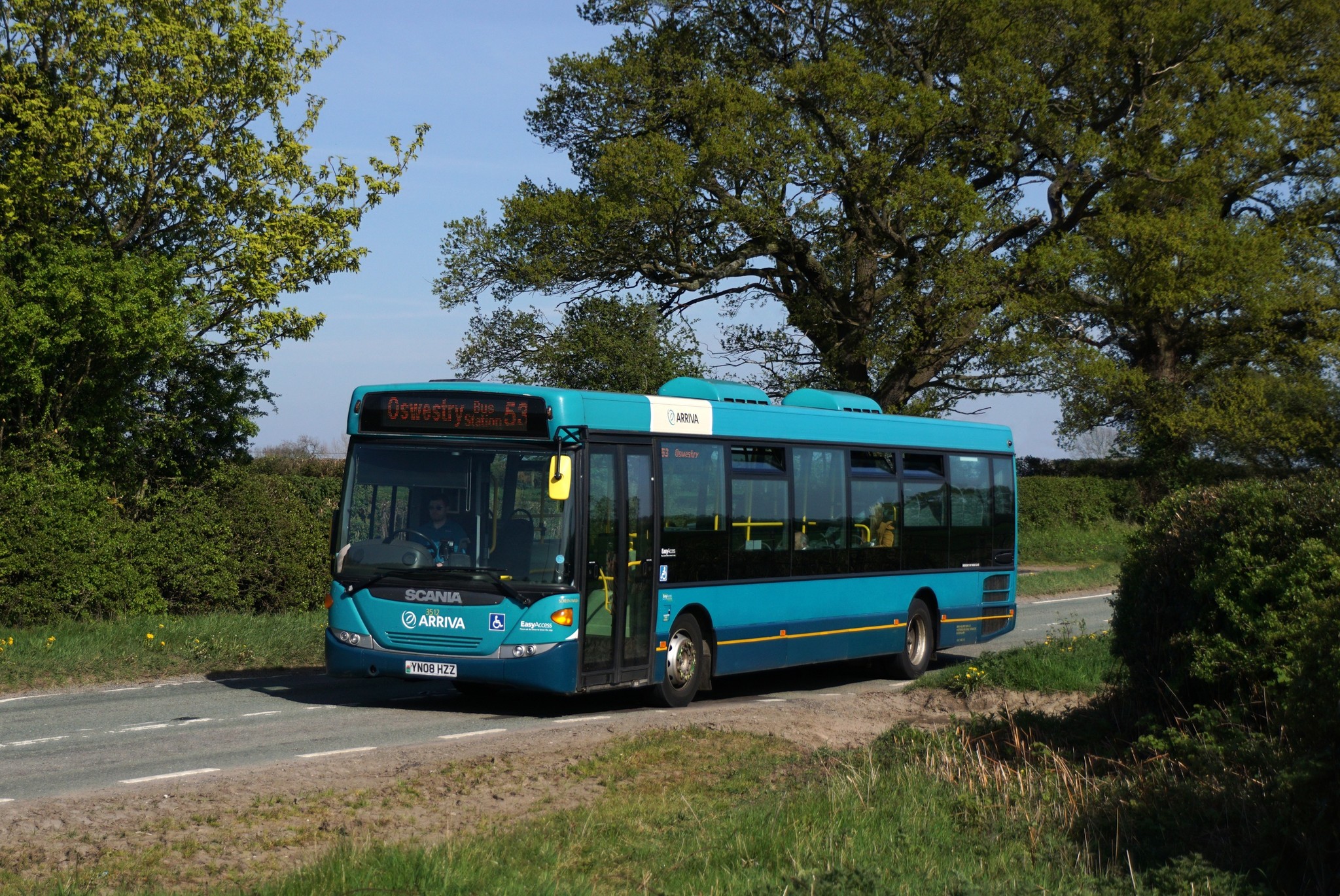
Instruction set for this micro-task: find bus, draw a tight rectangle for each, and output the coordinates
[322,376,1018,708]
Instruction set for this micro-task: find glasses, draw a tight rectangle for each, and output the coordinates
[428,504,445,511]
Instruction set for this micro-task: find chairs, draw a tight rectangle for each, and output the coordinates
[738,486,1004,550]
[447,506,533,578]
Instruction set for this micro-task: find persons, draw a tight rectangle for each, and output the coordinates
[410,493,468,556]
[793,532,813,550]
[858,500,895,547]
